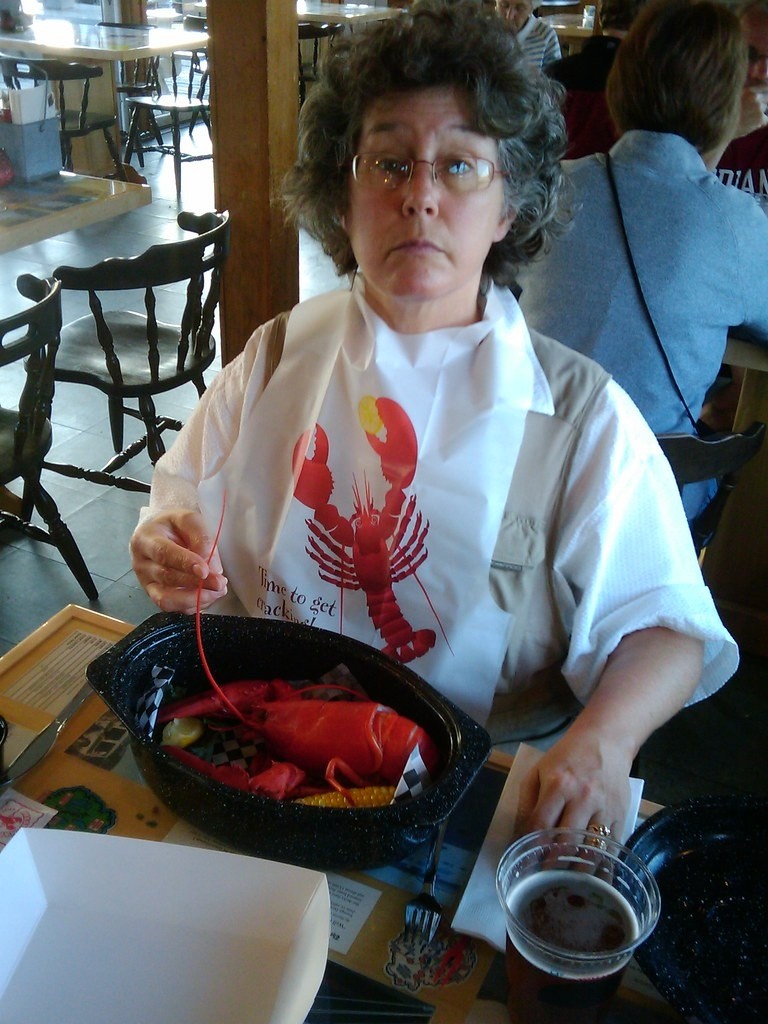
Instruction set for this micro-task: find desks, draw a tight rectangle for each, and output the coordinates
[297,0,404,70]
[0,19,210,188]
[0,170,153,518]
[536,13,628,55]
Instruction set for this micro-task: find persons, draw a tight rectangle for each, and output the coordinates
[512,0,768,547]
[128,0,739,884]
[494,0,562,76]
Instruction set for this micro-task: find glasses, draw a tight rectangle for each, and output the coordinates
[348,152,513,192]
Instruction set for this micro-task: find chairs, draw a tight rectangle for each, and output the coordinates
[24,210,232,493]
[0,52,129,185]
[0,274,99,601]
[97,23,163,169]
[124,13,213,192]
[656,420,768,564]
[298,21,347,108]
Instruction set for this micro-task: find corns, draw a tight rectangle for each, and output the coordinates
[289,785,398,809]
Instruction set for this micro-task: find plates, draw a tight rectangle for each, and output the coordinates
[612,791,768,1023]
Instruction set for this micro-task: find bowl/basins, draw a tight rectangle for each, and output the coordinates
[85,612,491,872]
[495,828,661,980]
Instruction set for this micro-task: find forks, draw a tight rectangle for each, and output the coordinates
[401,817,449,948]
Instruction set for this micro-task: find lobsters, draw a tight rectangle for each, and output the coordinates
[156,676,442,804]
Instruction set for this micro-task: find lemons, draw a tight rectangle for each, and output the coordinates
[161,717,204,749]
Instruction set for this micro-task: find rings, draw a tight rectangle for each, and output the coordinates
[585,825,610,857]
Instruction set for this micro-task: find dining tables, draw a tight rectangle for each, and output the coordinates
[1,605,673,1023]
